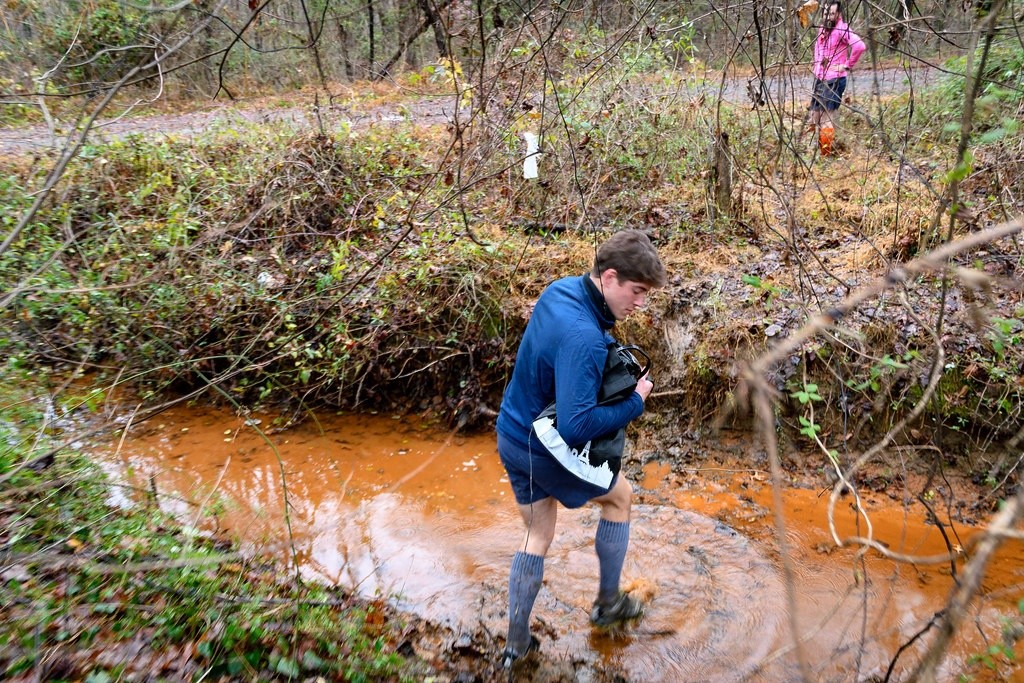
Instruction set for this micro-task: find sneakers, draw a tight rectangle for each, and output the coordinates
[590,591,645,626]
[502,636,540,667]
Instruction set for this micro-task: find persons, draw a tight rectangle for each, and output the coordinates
[811,0,866,156]
[495,229,665,668]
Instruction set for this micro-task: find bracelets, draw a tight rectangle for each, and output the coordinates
[844,66,849,71]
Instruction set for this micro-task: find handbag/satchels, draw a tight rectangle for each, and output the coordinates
[530,343,651,490]
